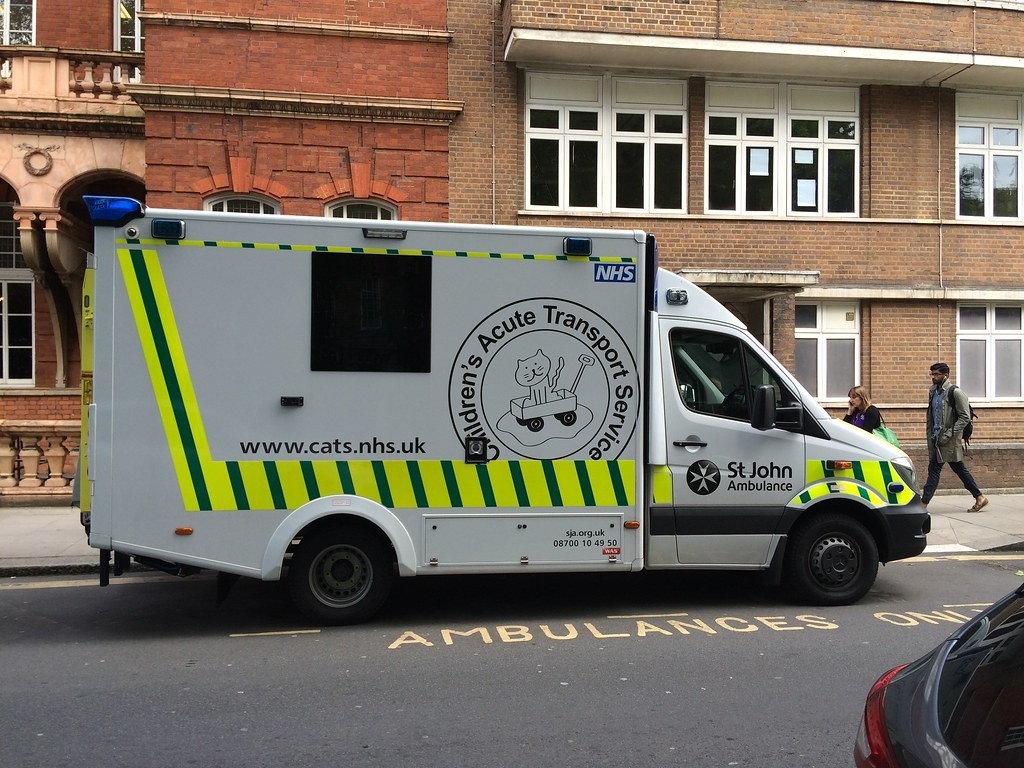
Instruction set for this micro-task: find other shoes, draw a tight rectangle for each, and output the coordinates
[967,498,989,513]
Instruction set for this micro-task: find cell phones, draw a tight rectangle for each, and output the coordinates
[849,401,853,406]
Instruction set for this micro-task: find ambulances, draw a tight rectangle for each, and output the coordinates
[73,194,932,629]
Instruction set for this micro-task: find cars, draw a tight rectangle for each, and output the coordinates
[851,583,1024,768]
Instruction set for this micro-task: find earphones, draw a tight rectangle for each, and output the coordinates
[943,376,947,380]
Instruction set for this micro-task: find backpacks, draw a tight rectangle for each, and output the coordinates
[948,385,978,452]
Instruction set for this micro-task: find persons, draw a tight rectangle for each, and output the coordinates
[842,386,881,434]
[920,363,989,512]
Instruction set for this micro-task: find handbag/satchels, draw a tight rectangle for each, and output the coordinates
[866,406,901,449]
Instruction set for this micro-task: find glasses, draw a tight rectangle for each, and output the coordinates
[929,372,945,376]
